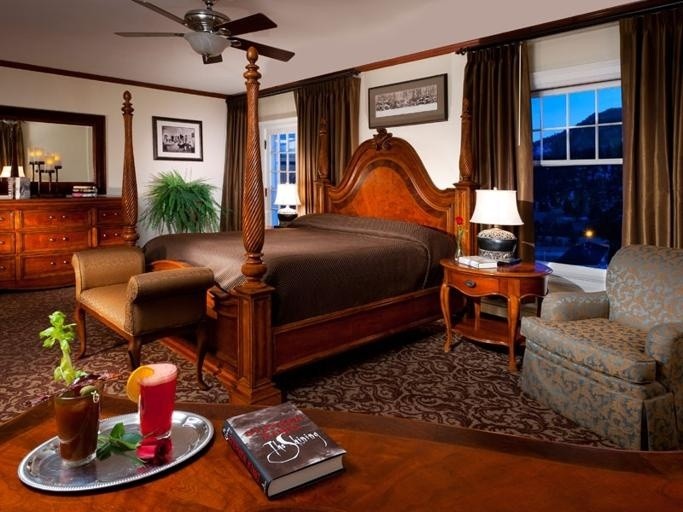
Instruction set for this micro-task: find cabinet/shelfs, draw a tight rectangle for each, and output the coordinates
[0,196,121,289]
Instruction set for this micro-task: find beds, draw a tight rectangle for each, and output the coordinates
[121,44,478,408]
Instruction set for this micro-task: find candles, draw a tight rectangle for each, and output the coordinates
[44,156,54,171]
[34,148,44,161]
[52,153,62,167]
[27,147,35,163]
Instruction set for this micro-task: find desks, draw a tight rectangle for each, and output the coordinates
[438,257,553,371]
[0,394,683,512]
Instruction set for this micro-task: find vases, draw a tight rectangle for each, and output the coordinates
[455,230,464,263]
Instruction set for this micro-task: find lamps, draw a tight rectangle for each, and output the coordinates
[183,33,231,57]
[0,165,26,177]
[274,184,303,222]
[469,186,524,264]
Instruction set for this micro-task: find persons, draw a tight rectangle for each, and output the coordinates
[162,134,194,152]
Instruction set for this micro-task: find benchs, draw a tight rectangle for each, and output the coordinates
[70,245,219,391]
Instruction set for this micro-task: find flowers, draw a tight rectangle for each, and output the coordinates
[455,216,466,255]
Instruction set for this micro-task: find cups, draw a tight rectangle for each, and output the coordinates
[53,382,106,468]
[138,363,179,441]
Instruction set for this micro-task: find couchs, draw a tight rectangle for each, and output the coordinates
[518,245,683,451]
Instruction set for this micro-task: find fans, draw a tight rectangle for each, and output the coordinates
[114,0,294,64]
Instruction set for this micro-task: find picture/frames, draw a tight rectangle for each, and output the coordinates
[152,116,203,162]
[368,73,448,129]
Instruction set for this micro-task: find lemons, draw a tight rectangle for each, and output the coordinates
[126,366,154,404]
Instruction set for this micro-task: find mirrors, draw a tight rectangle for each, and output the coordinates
[0,105,106,197]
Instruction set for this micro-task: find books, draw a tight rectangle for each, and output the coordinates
[456,255,497,268]
[8,177,30,200]
[72,185,98,198]
[221,400,347,498]
